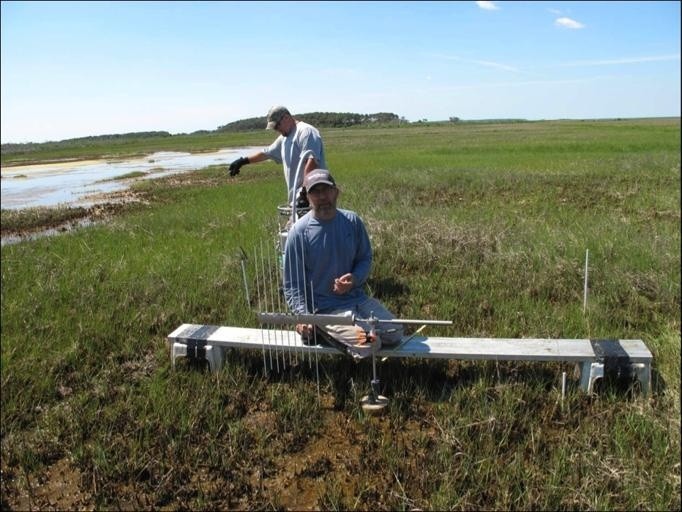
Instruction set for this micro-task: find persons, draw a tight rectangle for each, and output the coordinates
[228,106,327,219]
[280,166,403,396]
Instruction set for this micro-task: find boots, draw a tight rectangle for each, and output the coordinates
[300,322,349,358]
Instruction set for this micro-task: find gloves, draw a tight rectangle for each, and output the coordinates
[294,186,311,209]
[228,156,251,178]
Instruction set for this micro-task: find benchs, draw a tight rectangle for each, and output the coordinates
[167,323,655,399]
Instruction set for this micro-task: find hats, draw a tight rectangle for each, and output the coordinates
[301,168,336,194]
[264,106,290,130]
